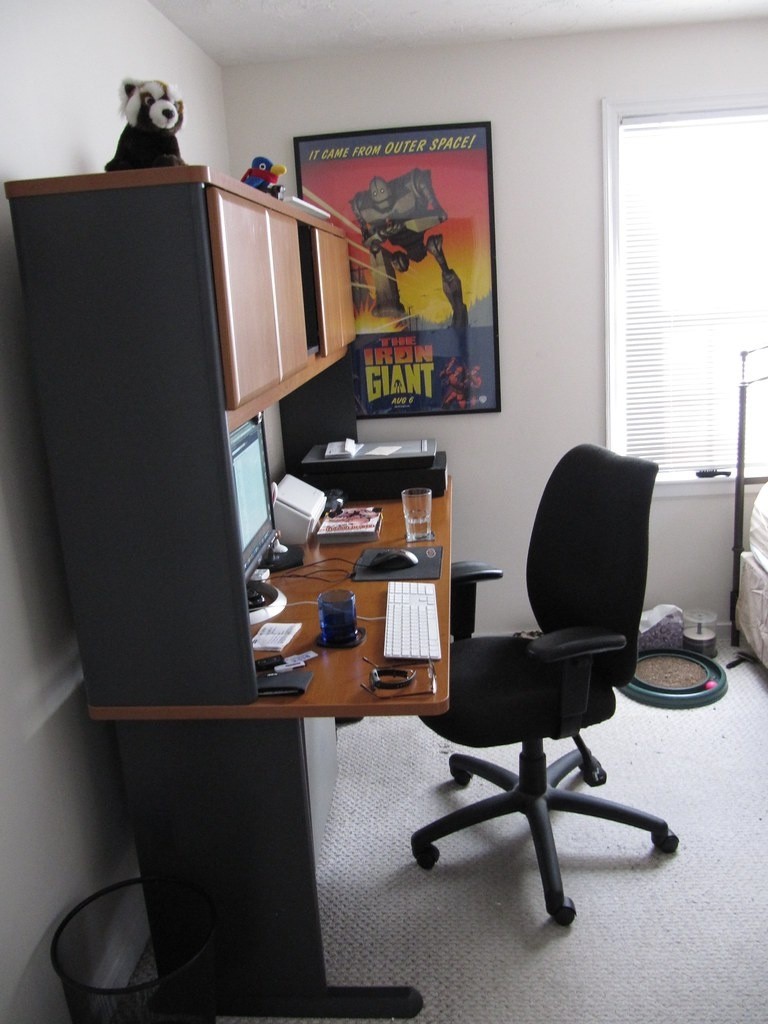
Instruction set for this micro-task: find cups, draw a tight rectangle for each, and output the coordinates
[318,589,359,643]
[401,488,432,540]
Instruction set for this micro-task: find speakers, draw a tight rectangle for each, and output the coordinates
[272,474,327,549]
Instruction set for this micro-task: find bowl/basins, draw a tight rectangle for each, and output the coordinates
[634,654,710,693]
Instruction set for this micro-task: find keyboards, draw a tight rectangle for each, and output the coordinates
[383,581,441,660]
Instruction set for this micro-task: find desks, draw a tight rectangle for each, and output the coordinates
[89,473,453,1020]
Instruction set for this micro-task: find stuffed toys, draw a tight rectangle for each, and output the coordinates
[241,157,287,201]
[105,80,183,172]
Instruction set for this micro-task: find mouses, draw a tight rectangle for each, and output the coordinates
[370,549,418,571]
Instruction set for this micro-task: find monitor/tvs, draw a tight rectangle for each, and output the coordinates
[228,411,279,613]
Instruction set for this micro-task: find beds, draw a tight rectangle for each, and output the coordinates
[734,482,768,669]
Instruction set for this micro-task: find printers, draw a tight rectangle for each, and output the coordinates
[301,439,448,499]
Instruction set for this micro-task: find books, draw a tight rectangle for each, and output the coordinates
[317,504,384,544]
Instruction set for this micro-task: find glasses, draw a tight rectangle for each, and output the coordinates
[360,656,437,699]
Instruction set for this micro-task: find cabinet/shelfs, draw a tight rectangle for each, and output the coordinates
[6,167,357,706]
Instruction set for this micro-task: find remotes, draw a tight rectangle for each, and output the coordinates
[696,470,731,477]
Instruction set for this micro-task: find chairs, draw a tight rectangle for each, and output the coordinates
[410,444,680,924]
